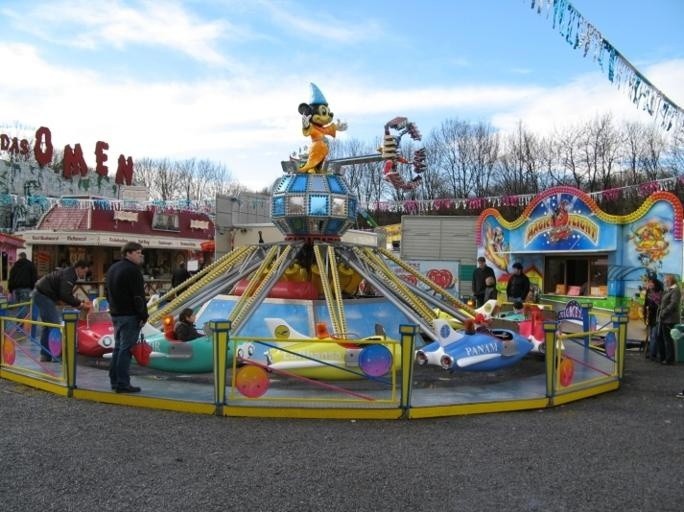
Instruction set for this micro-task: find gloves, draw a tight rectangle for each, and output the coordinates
[77,300,93,311]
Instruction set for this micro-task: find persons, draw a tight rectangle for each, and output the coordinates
[642,275,681,364]
[32,259,92,362]
[504,263,531,302]
[191,260,208,276]
[7,251,37,331]
[174,307,198,343]
[104,242,150,393]
[511,301,525,316]
[471,256,496,306]
[474,276,498,305]
[171,260,193,296]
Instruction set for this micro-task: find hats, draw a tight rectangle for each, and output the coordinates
[648,272,658,279]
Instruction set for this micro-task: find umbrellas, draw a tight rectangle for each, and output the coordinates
[128,333,154,368]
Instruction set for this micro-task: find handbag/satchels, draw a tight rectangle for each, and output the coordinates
[131,340,152,365]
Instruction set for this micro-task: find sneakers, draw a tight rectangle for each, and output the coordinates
[39,353,54,361]
[112,380,142,393]
[658,359,674,364]
